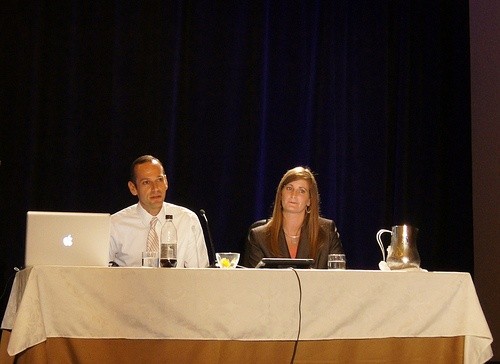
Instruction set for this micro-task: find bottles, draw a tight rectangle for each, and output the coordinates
[160,215,178,268]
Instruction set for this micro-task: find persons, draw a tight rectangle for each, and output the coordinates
[246,167,345,269]
[108,155,211,269]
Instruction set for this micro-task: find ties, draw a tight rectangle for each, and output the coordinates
[146,217,159,268]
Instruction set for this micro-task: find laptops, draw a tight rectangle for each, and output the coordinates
[25,212,112,266]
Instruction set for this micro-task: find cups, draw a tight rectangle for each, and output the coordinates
[142,251,159,267]
[327,254,346,269]
[215,253,240,269]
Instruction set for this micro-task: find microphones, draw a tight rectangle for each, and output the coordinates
[199,210,218,269]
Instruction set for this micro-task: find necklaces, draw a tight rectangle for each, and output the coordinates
[284,229,300,238]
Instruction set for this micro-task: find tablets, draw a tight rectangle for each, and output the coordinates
[260,257,315,268]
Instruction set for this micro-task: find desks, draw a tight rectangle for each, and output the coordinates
[0,264,493,364]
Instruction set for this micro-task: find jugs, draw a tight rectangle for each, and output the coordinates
[376,225,421,270]
